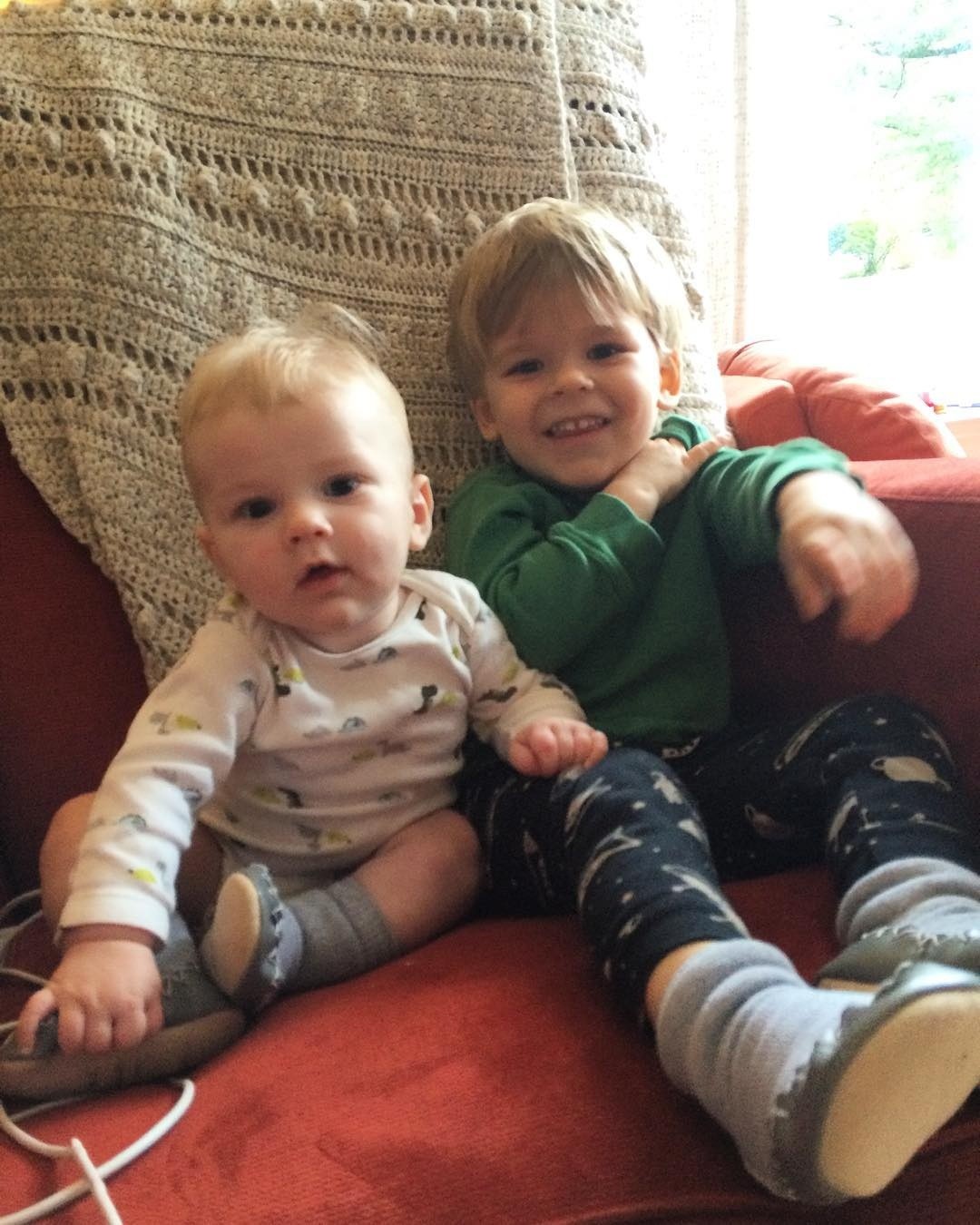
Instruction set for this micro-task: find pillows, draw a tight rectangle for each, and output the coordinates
[716,338,965,462]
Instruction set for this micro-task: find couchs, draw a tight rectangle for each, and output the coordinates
[0,0,980,1225]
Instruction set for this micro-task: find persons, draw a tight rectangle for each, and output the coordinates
[441,194,979,1208]
[0,301,609,1102]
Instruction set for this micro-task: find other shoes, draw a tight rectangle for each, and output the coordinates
[0,942,243,1101]
[818,926,980,996]
[778,964,979,1210]
[200,861,280,1011]
